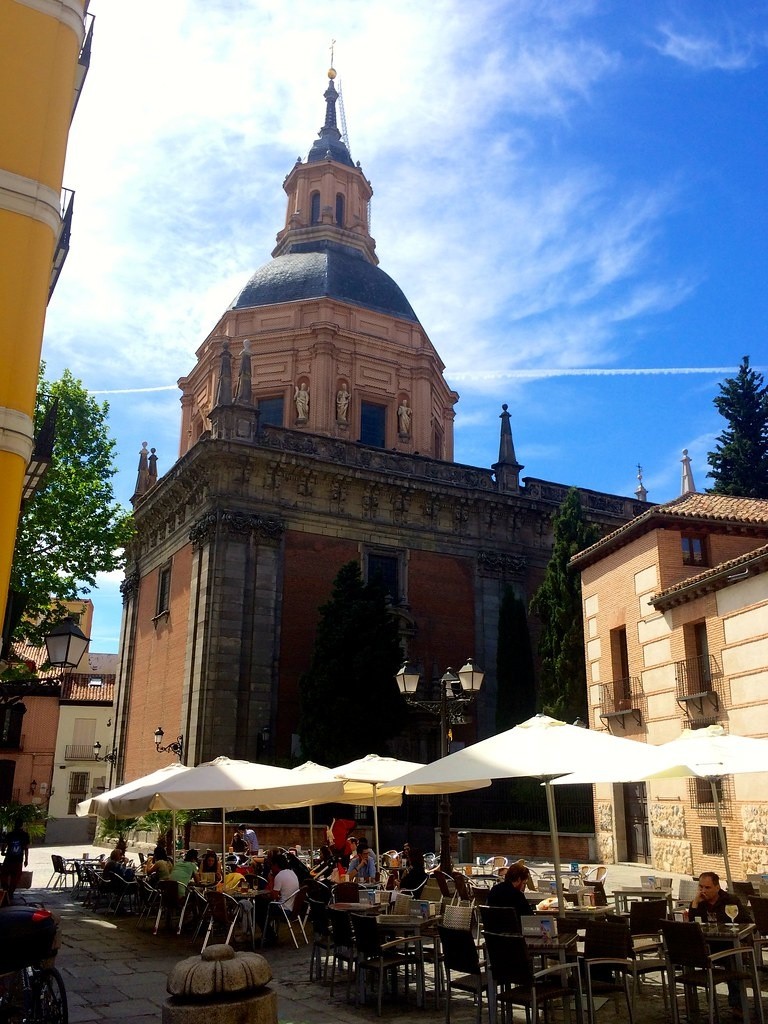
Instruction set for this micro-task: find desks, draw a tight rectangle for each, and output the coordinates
[658,923,759,1024]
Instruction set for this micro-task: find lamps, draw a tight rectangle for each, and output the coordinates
[30,779,37,795]
[0,616,89,715]
[256,726,271,746]
[573,717,587,729]
[154,727,183,761]
[93,741,117,768]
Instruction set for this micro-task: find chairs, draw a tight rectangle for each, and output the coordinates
[45,850,767,1024]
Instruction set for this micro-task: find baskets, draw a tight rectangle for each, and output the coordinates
[394,893,413,915]
[443,894,475,931]
[376,891,391,904]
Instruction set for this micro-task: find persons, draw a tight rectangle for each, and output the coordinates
[101,822,427,948]
[0,817,29,904]
[397,398,413,435]
[485,863,534,927]
[685,872,759,1021]
[293,382,310,419]
[337,382,351,421]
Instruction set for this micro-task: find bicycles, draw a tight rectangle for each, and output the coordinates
[0,895,68,1024]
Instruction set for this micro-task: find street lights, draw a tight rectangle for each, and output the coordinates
[392,657,486,872]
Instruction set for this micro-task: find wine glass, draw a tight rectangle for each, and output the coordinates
[253,879,259,893]
[725,905,740,932]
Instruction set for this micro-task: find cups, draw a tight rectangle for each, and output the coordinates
[83,853,89,859]
[694,917,702,924]
[566,902,574,908]
[258,849,263,857]
[241,881,250,894]
[583,896,591,906]
[295,844,301,854]
[707,912,717,927]
[200,872,216,884]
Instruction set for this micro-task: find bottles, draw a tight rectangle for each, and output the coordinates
[143,864,147,874]
[307,848,312,858]
[226,846,237,873]
[132,863,136,873]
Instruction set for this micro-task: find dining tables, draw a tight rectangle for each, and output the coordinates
[541,871,581,884]
[612,888,673,921]
[382,866,406,891]
[235,890,272,934]
[461,875,500,889]
[329,903,390,933]
[452,863,485,887]
[379,914,443,1007]
[330,883,381,905]
[485,933,578,1024]
[532,906,616,956]
[65,859,101,889]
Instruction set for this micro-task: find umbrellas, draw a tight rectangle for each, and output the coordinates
[72,712,494,870]
[377,712,661,920]
[541,725,768,894]
